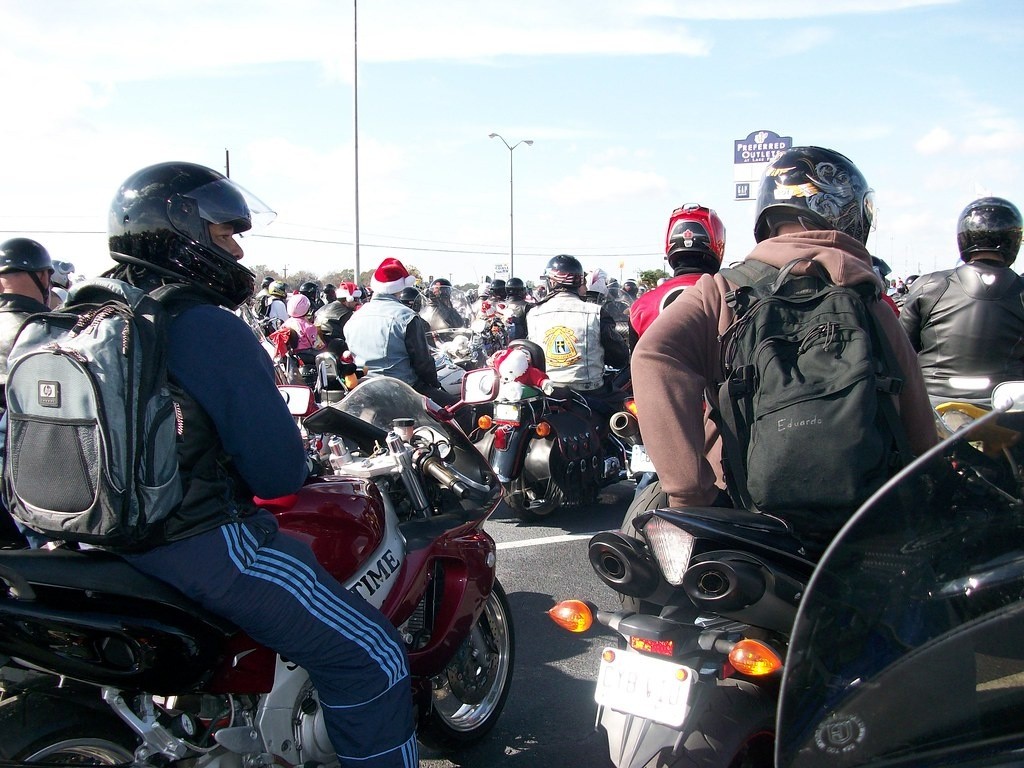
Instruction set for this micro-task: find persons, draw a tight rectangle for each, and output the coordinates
[628,204,727,356]
[0,237,76,408]
[400,267,671,361]
[630,146,940,508]
[870,255,921,316]
[899,197,1024,408]
[342,258,473,437]
[52,162,419,768]
[528,256,629,416]
[252,276,374,352]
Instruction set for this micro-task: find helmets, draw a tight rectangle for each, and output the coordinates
[48,259,76,289]
[299,281,318,296]
[665,201,727,273]
[269,279,288,297]
[620,282,639,295]
[752,145,878,249]
[505,278,524,296]
[488,279,506,290]
[539,254,585,289]
[108,161,280,312]
[0,238,56,275]
[956,197,1023,266]
[400,287,422,313]
[429,278,452,289]
[323,283,337,296]
[261,276,274,288]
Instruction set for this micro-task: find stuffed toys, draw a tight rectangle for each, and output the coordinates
[486,346,554,402]
[436,335,468,358]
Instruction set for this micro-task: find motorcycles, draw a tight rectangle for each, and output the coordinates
[0,367,516,768]
[542,404,1024,768]
[255,313,375,468]
[481,339,647,517]
[605,396,733,625]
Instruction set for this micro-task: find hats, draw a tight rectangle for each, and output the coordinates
[607,278,622,289]
[370,256,415,295]
[287,294,311,317]
[335,282,362,303]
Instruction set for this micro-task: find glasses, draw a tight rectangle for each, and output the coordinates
[354,297,360,302]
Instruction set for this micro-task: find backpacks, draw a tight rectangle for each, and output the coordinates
[5,277,205,553]
[252,295,272,320]
[716,261,938,550]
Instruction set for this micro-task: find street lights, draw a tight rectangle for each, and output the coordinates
[489,132,533,280]
